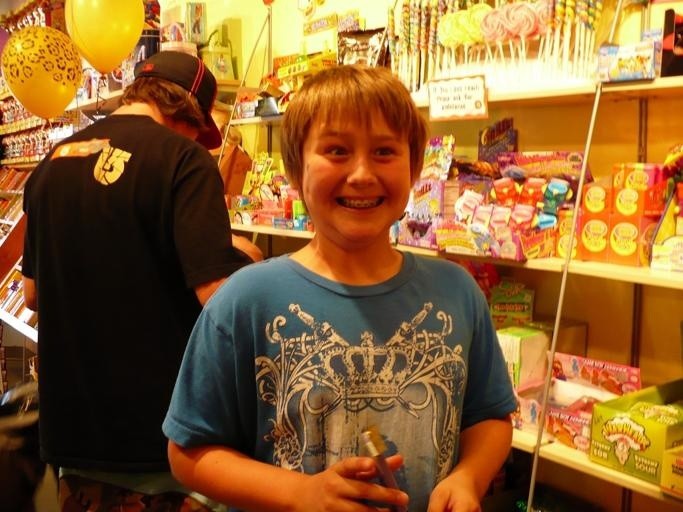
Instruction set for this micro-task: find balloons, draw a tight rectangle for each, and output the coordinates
[64,0,145,74]
[0,26,82,119]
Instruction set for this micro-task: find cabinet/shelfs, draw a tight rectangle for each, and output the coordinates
[0,89,126,169]
[230,76,683,512]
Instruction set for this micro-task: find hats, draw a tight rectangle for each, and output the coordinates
[135,52,222,150]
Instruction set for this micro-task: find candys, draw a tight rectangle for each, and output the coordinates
[384,1,602,94]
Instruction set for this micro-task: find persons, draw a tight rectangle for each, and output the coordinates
[21,51,264,512]
[160,67,519,512]
